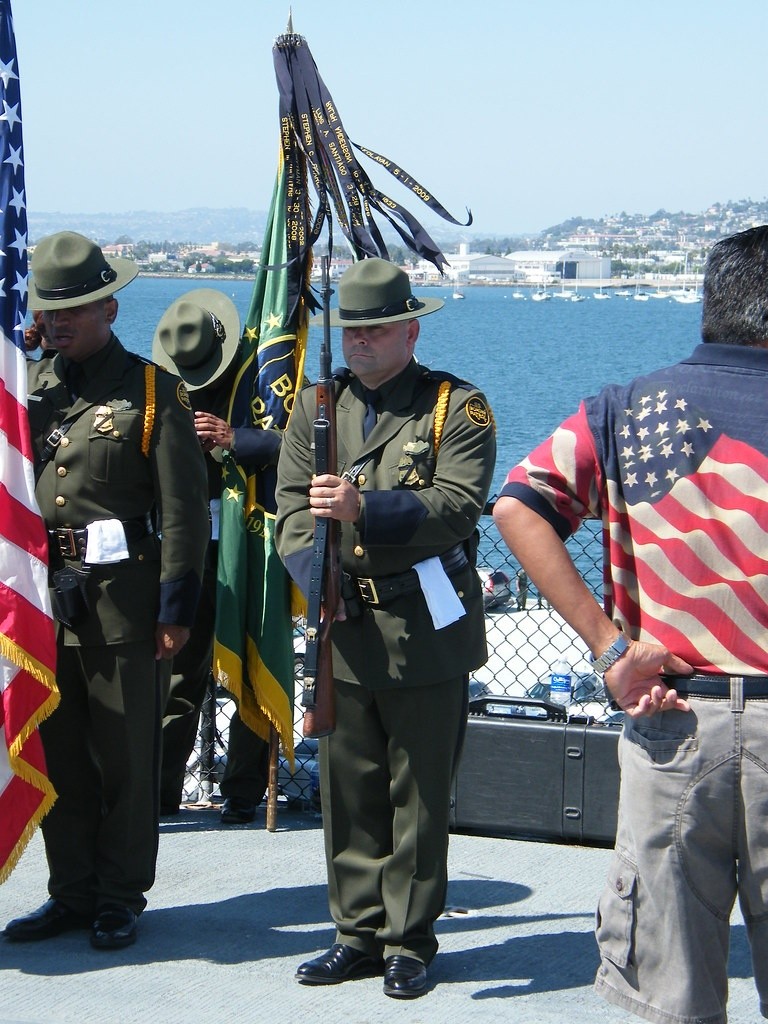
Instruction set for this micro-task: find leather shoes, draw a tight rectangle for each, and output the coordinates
[4,898,92,940]
[294,944,384,983]
[382,955,427,995]
[218,797,257,823]
[90,904,137,948]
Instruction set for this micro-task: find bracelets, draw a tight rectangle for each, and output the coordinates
[588,631,632,674]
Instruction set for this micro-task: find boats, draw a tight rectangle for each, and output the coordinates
[615,291,632,296]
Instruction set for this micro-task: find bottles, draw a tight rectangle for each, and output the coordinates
[550,655,572,724]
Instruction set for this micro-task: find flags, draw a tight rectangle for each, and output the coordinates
[210,161,303,774]
[0,0,62,888]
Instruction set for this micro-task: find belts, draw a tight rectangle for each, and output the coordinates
[658,670,767,700]
[343,541,471,606]
[47,512,154,560]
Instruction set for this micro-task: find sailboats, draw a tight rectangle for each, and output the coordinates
[571,262,586,303]
[634,254,649,301]
[452,276,465,299]
[592,260,611,299]
[512,249,551,301]
[646,252,704,303]
[553,261,575,298]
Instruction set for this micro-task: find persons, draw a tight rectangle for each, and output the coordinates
[493,222,768,1023]
[515,565,530,612]
[536,591,551,609]
[273,257,498,1000]
[154,286,312,828]
[5,230,212,952]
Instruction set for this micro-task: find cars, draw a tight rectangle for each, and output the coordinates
[478,568,510,612]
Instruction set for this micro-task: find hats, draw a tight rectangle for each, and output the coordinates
[151,287,241,391]
[26,230,139,310]
[307,257,445,327]
